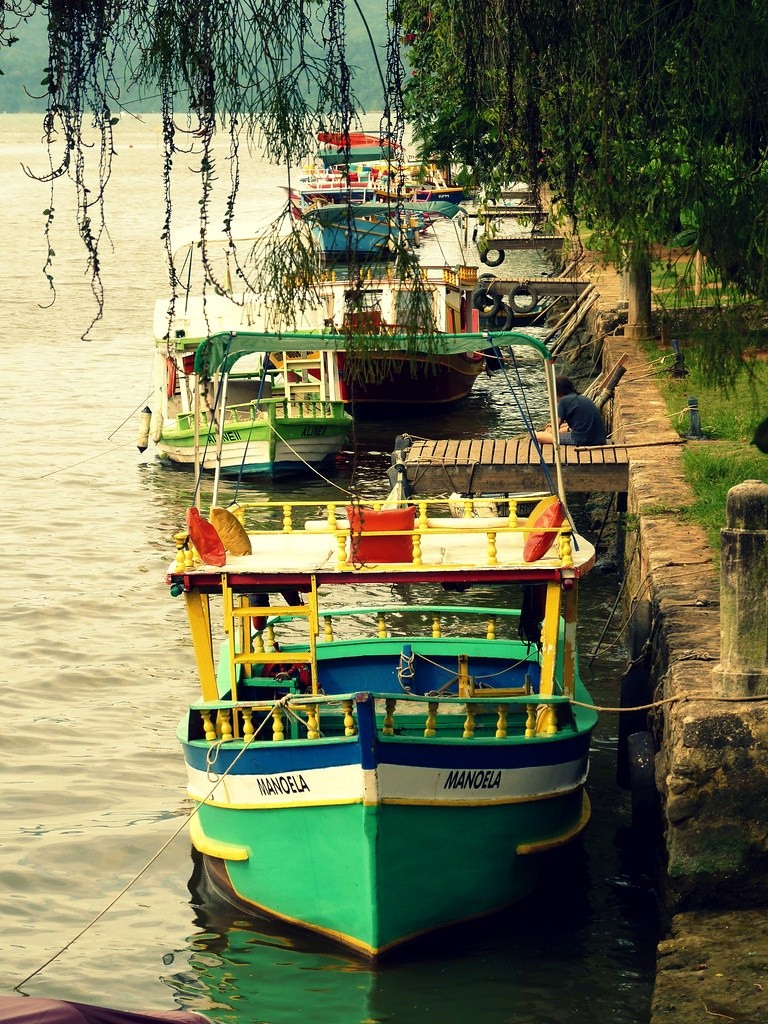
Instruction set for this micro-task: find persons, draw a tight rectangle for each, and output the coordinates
[536,375,606,445]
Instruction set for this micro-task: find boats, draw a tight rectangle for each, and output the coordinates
[150,300,352,482]
[165,330,599,964]
[279,130,477,269]
[270,266,486,406]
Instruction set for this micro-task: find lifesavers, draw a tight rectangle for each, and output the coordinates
[482,246,506,267]
[473,283,538,333]
